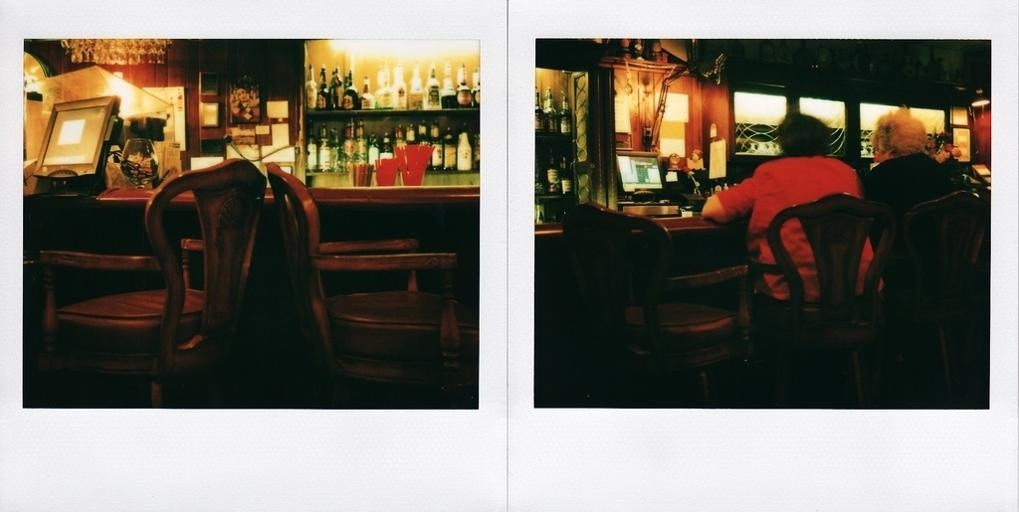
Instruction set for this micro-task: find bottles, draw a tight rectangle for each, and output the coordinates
[688,170,706,197]
[306,119,479,171]
[593,38,663,62]
[535,88,570,135]
[535,199,545,224]
[535,146,572,197]
[304,59,481,109]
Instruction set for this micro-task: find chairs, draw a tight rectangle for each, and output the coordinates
[267,164,478,409]
[564,202,748,407]
[897,190,989,409]
[737,193,897,409]
[36,162,266,408]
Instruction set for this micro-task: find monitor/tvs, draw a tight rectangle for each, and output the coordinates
[615,150,664,203]
[30,94,123,195]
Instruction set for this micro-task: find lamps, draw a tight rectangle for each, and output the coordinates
[971,89,990,108]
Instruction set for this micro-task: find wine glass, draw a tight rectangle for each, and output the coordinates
[66,40,169,65]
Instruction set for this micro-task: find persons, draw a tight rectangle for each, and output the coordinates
[865,107,979,315]
[701,112,885,322]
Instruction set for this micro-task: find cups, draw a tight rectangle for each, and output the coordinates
[118,137,160,190]
[393,143,435,185]
[374,158,397,186]
[352,164,372,187]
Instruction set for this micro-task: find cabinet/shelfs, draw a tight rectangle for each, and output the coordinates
[534,129,574,237]
[305,108,480,177]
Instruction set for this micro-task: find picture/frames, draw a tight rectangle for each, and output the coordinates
[226,70,262,127]
